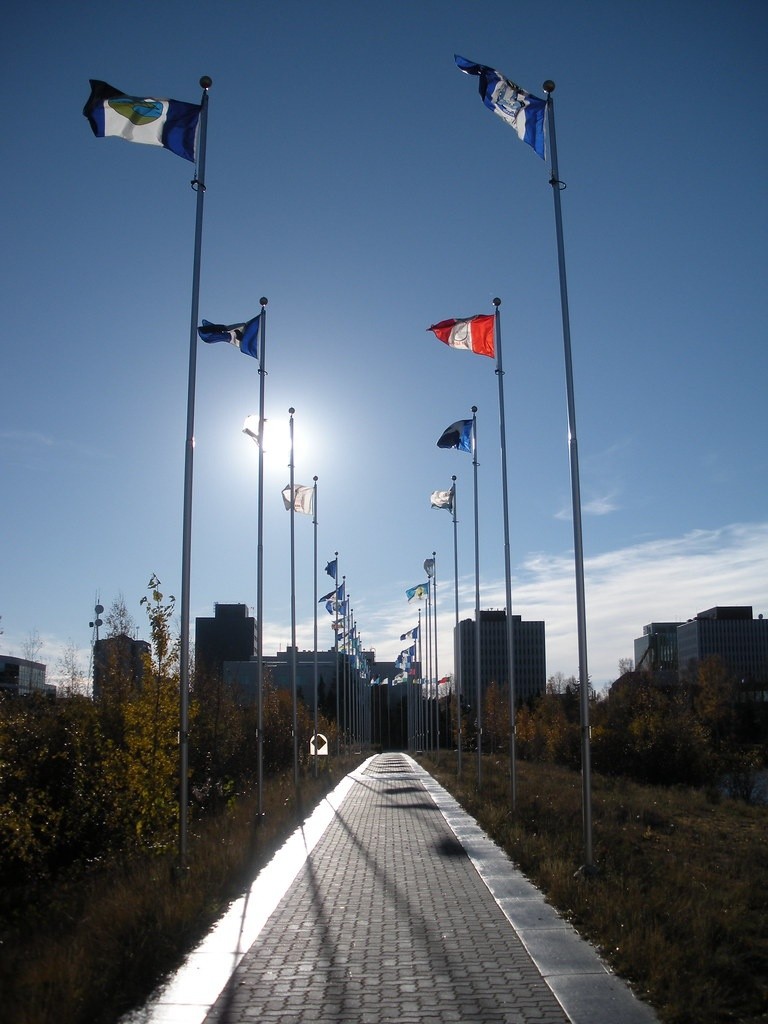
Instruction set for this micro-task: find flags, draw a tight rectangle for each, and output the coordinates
[452,54,546,160]
[427,314,495,359]
[83,79,202,161]
[430,487,454,514]
[199,314,261,359]
[283,483,314,514]
[319,559,451,688]
[437,419,474,454]
